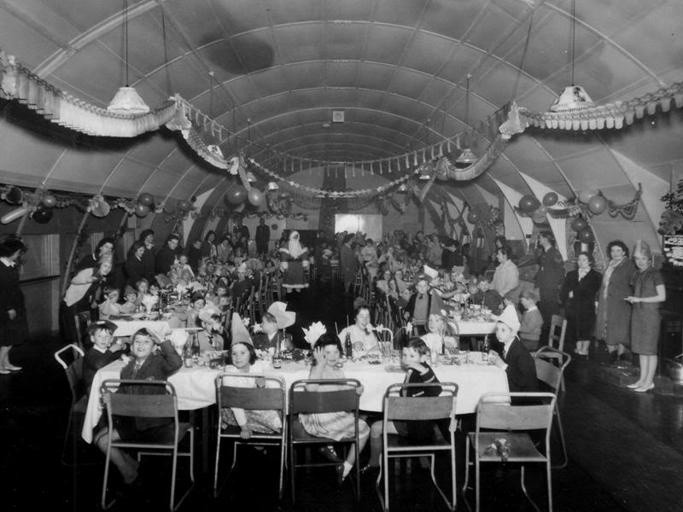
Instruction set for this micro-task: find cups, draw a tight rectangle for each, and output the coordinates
[341,341,401,365]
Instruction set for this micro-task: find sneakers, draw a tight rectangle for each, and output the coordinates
[319,446,339,461]
[336,464,344,488]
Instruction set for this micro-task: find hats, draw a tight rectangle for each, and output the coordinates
[413,273,432,284]
[430,290,441,314]
[231,312,253,347]
[87,320,117,330]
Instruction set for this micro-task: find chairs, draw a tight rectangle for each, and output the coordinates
[100,378,196,509]
[289,378,365,505]
[462,391,558,510]
[374,382,459,511]
[213,372,287,500]
[54,344,90,443]
[534,344,572,469]
[56,255,572,359]
[529,315,568,392]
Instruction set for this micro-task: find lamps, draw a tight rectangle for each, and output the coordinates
[550,0,596,112]
[208,71,292,199]
[397,119,431,192]
[107,0,150,115]
[457,73,477,164]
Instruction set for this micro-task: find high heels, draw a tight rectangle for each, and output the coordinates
[626,382,655,392]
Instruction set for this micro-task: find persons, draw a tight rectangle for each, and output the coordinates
[1,189,665,486]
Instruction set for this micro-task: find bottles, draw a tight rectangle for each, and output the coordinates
[434,333,492,368]
[135,289,184,320]
[459,294,492,322]
[271,331,284,370]
[184,328,201,370]
[344,334,351,357]
